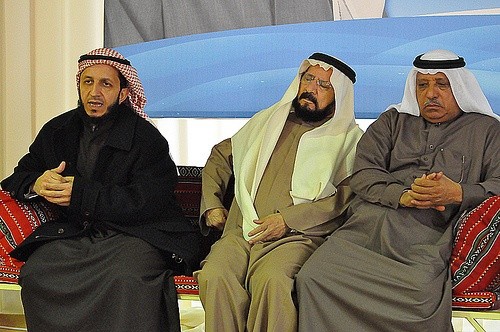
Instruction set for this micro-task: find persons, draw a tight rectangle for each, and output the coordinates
[193,52,366,332]
[297,50,500,332]
[0,49,218,332]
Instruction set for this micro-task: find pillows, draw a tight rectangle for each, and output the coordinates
[1,167,499,313]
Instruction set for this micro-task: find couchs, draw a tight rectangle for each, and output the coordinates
[0,114,499,331]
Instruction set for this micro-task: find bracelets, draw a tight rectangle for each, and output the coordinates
[400,190,408,208]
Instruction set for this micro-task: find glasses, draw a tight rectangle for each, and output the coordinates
[299,73,333,91]
